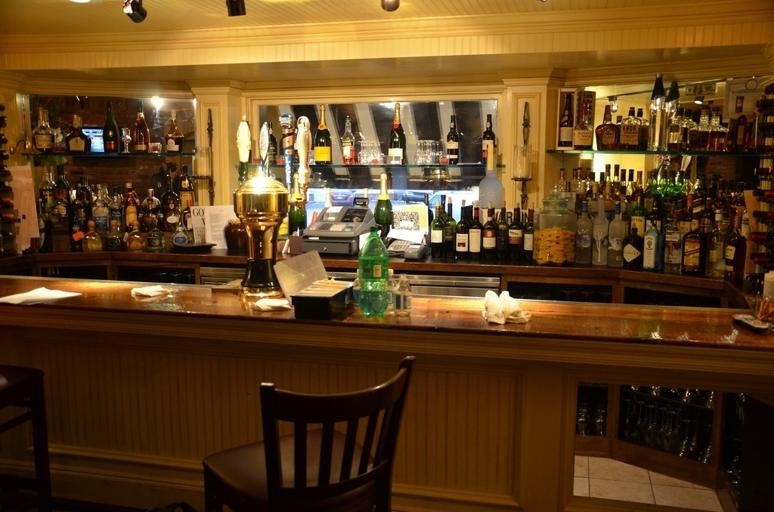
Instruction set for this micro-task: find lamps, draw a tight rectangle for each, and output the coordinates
[121,0,147,23]
[694,96,704,104]
[381,0,400,11]
[226,0,246,16]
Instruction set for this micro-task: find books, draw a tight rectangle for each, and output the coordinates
[191,206,205,244]
[203,206,238,249]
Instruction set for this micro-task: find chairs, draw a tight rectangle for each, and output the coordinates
[201,354,415,511]
[1,364,53,511]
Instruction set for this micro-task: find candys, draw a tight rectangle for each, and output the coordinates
[536,227,574,266]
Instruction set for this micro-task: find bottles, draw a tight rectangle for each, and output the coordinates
[617,169,629,198]
[667,108,688,152]
[582,167,591,187]
[144,190,160,208]
[481,114,496,164]
[342,116,355,164]
[64,115,87,153]
[508,204,524,264]
[599,164,614,203]
[662,172,678,217]
[124,191,139,232]
[423,195,433,226]
[177,166,195,208]
[705,176,753,209]
[614,115,622,129]
[608,202,629,268]
[265,123,275,163]
[289,176,305,238]
[70,188,89,233]
[678,172,688,193]
[160,178,180,231]
[568,168,584,194]
[705,209,726,279]
[496,201,508,264]
[43,109,57,151]
[114,185,124,205]
[37,166,52,210]
[680,109,697,151]
[395,274,410,315]
[586,173,598,212]
[387,103,406,165]
[55,166,68,200]
[533,189,576,266]
[386,269,397,315]
[166,119,182,153]
[374,174,394,239]
[725,207,747,282]
[619,107,641,149]
[356,228,388,317]
[107,188,122,231]
[612,164,620,197]
[430,203,443,258]
[481,202,496,263]
[455,200,468,258]
[32,108,55,152]
[688,107,713,153]
[77,116,91,151]
[127,187,140,201]
[443,198,456,258]
[574,200,592,265]
[573,103,592,149]
[571,167,576,179]
[664,81,681,122]
[99,184,112,203]
[523,208,535,263]
[167,209,190,249]
[706,108,728,152]
[102,100,120,152]
[106,220,123,250]
[83,221,102,249]
[645,193,662,234]
[314,106,332,164]
[479,140,506,226]
[591,199,609,266]
[595,105,617,149]
[468,206,483,259]
[77,177,92,200]
[634,108,646,149]
[91,186,108,228]
[688,176,706,218]
[446,115,460,164]
[558,95,573,146]
[631,171,644,216]
[124,221,145,249]
[71,222,84,251]
[643,215,662,272]
[134,100,150,151]
[682,215,705,277]
[622,228,643,271]
[142,199,160,232]
[440,195,446,215]
[626,169,636,197]
[646,72,667,151]
[552,169,568,195]
[643,170,659,214]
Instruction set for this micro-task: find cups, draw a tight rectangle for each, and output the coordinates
[394,278,411,318]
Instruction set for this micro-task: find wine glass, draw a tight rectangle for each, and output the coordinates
[415,140,442,163]
[357,140,382,164]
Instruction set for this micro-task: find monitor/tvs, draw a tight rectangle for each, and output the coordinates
[82,127,105,153]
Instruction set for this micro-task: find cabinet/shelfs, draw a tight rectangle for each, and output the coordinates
[0,10,774,311]
[0,250,772,310]
[1,275,774,512]
